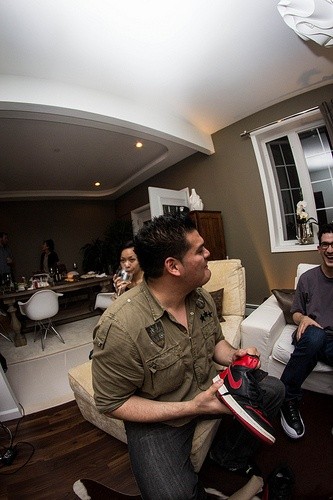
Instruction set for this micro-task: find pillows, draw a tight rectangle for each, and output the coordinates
[208,287,226,322]
[270,288,299,326]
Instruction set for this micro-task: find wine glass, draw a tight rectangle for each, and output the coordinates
[111,269,133,301]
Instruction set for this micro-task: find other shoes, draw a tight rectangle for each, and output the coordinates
[203,487,230,500]
[210,452,260,478]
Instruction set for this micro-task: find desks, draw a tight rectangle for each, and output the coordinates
[0,273,114,347]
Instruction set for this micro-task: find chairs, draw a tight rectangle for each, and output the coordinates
[17,289,65,353]
[94,293,116,313]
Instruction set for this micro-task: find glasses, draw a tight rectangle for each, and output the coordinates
[320,242,333,250]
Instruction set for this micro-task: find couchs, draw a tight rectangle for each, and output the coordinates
[67,259,246,474]
[240,262,333,396]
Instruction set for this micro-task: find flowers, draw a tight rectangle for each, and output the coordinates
[294,191,318,224]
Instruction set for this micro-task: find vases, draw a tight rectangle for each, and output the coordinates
[296,221,318,245]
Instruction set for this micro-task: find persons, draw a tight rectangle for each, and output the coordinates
[40,239,59,273]
[113,241,143,300]
[280,223,333,438]
[89,210,286,500]
[0,232,13,273]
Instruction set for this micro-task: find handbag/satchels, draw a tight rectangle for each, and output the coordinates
[55,265,67,275]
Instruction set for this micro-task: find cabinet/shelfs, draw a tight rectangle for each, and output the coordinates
[183,209,228,262]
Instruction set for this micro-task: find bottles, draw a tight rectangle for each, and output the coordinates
[54,268,62,281]
[49,268,56,282]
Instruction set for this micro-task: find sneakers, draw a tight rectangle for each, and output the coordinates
[280,400,305,439]
[262,462,295,500]
[212,353,279,446]
